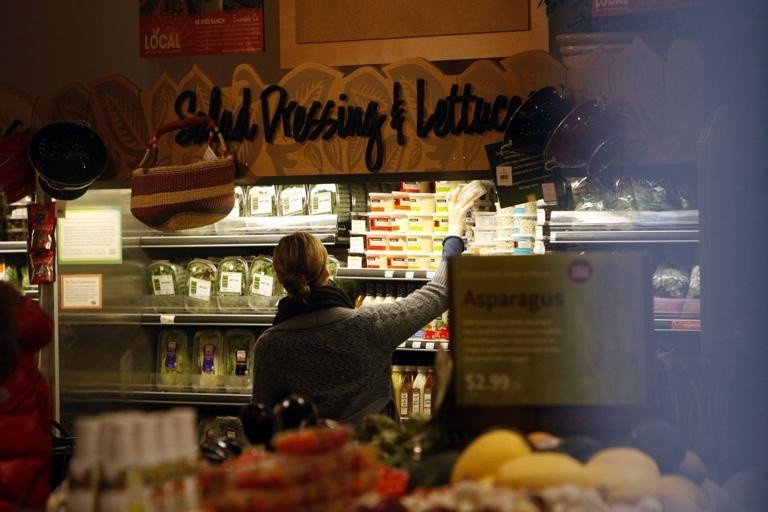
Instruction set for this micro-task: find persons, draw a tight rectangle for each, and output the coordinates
[254,185,486,446]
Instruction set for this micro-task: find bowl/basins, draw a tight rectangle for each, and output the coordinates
[466,180,492,211]
[514,213,538,233]
[471,226,495,242]
[474,212,495,227]
[494,249,514,256]
[493,239,516,250]
[514,234,535,255]
[494,214,513,226]
[493,225,518,240]
[471,242,495,256]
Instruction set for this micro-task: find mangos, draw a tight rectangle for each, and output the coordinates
[453,429,710,511]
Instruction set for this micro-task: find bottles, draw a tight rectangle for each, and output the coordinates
[353,289,407,309]
[412,367,428,416]
[391,365,405,417]
[423,368,435,415]
[400,366,416,417]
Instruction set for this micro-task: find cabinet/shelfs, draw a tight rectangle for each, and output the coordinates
[277,0,551,70]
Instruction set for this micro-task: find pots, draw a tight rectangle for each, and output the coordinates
[500,82,626,178]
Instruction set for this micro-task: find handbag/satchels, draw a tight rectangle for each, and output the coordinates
[129,118,236,232]
[0,129,36,191]
